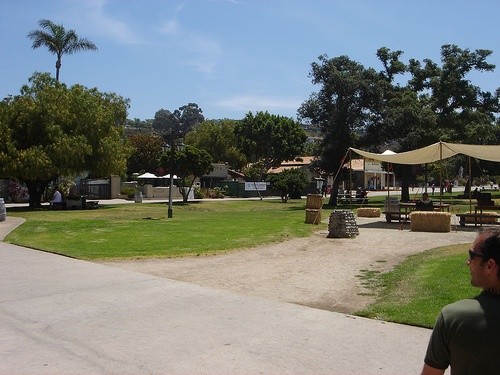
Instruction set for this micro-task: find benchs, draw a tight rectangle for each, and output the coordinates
[336,197,370,207]
[382,211,411,224]
[456,213,500,226]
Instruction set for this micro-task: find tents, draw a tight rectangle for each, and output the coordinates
[348,140,500,214]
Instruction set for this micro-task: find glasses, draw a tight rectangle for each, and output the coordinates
[468,249,497,263]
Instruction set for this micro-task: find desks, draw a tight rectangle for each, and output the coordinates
[338,193,363,205]
[397,203,449,224]
[474,205,500,227]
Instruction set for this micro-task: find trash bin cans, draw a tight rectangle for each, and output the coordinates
[0,198,6,221]
[134,192,142,203]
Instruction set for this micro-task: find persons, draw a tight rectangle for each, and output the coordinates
[420,227,500,375]
[441,177,452,195]
[336,186,351,199]
[355,186,367,198]
[489,181,493,187]
[50,187,63,203]
[459,166,463,176]
[464,180,468,194]
[431,183,435,193]
[320,182,333,198]
[416,192,434,211]
